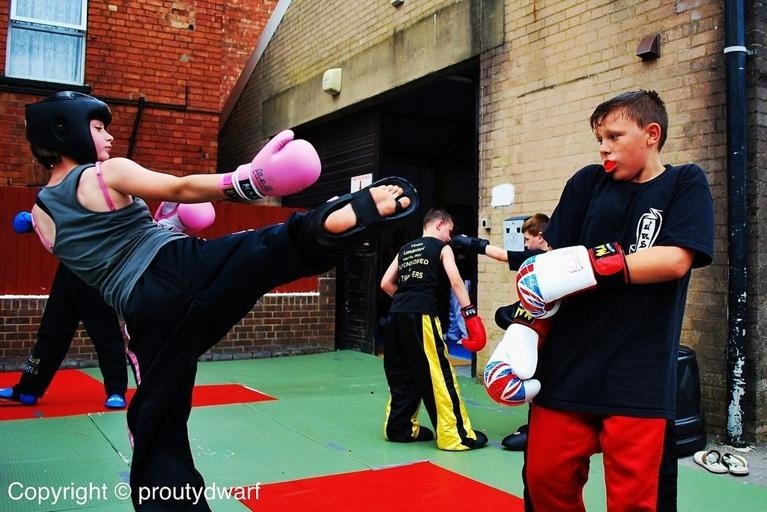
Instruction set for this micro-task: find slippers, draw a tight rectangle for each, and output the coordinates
[694,449,727,474]
[722,453,749,476]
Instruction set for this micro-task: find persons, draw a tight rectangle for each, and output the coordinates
[25,91,419,512]
[380,208,489,451]
[449,214,550,452]
[482,88,715,512]
[0,211,128,407]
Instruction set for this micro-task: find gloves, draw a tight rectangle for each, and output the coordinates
[150,201,215,237]
[516,241,630,321]
[482,301,554,407]
[219,129,322,204]
[13,211,34,235]
[449,233,490,255]
[460,303,486,352]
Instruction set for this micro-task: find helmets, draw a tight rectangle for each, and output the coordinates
[23,91,111,168]
